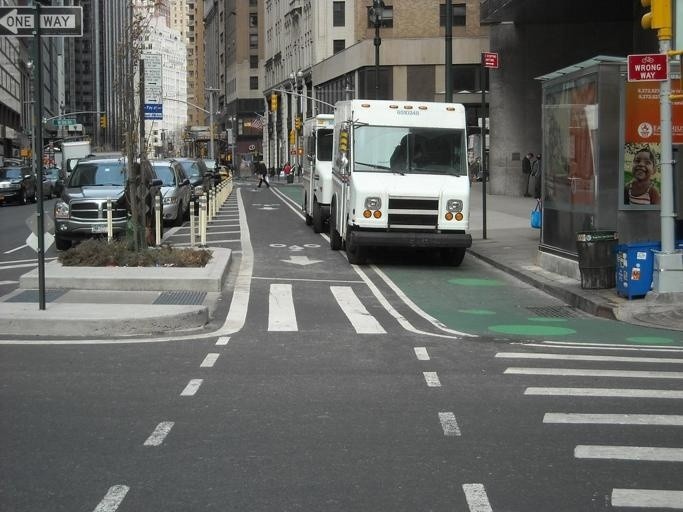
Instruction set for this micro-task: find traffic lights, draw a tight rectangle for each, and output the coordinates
[295,116,300,130]
[290,130,295,144]
[270,94,278,111]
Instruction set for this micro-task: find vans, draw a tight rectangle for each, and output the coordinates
[301,100,470,266]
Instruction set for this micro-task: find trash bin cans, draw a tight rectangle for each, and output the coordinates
[577,231,617,290]
[287,174,293,183]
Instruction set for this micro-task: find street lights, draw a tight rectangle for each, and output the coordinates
[289,69,303,183]
[367,0,389,99]
[162,86,220,151]
[22,100,109,151]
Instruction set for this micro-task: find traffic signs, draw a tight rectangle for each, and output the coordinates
[0,5,83,36]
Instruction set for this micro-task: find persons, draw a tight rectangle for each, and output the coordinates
[283,162,291,174]
[522,153,541,199]
[253,163,270,188]
[624,149,660,205]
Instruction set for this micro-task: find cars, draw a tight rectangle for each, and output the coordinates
[0,152,218,251]
